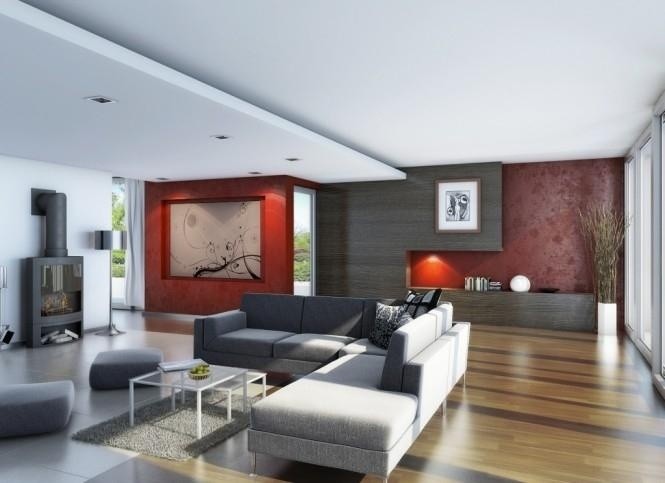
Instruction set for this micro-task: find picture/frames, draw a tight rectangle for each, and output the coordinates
[435,177,482,234]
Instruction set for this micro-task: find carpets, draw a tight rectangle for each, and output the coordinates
[69,393,284,462]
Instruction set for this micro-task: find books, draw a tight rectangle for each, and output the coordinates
[157,358,209,372]
[464,276,503,292]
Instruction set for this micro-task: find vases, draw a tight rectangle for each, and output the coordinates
[597,302,618,335]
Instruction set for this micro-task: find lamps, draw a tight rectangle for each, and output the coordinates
[94,230,128,337]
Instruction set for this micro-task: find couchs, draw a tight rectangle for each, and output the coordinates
[193,293,471,483]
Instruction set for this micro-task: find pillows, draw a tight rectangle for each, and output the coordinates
[404,289,443,319]
[369,300,405,351]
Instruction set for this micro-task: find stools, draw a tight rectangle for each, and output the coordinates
[89,348,163,392]
[1,380,74,438]
